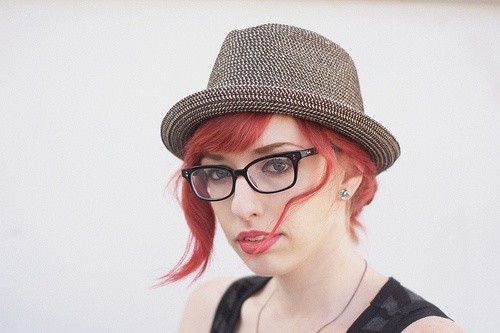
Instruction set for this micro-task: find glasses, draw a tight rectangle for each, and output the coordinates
[181,144,338,200]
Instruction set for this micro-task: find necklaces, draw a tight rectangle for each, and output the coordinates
[255,256,369,333]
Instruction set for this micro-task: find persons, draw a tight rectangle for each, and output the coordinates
[179,23,467,333]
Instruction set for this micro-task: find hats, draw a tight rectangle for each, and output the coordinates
[160,23,401,174]
